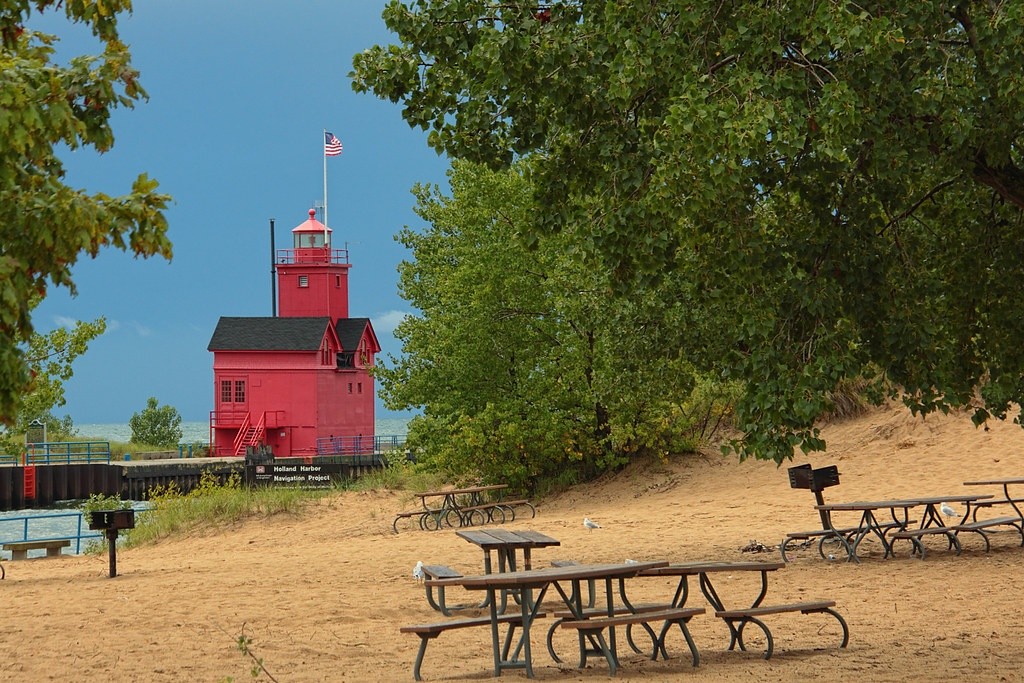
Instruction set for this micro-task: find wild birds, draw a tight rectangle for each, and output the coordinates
[412,561,425,584]
[940,502,963,520]
[584,518,602,534]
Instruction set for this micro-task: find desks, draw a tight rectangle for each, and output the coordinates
[413,561,700,683]
[424,485,535,531]
[393,488,506,534]
[425,528,595,618]
[882,494,1024,555]
[781,502,962,563]
[546,560,849,662]
[963,478,1024,531]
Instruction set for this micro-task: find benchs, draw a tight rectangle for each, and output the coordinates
[951,516,1022,531]
[400,612,547,633]
[497,499,528,506]
[786,528,865,537]
[423,577,464,586]
[888,526,953,538]
[551,561,578,567]
[715,600,837,618]
[561,607,707,629]
[396,505,465,516]
[961,498,1024,506]
[861,519,918,531]
[553,602,672,618]
[2,540,71,560]
[461,503,497,512]
[421,564,463,580]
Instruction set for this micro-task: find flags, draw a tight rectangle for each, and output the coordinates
[325,132,343,156]
